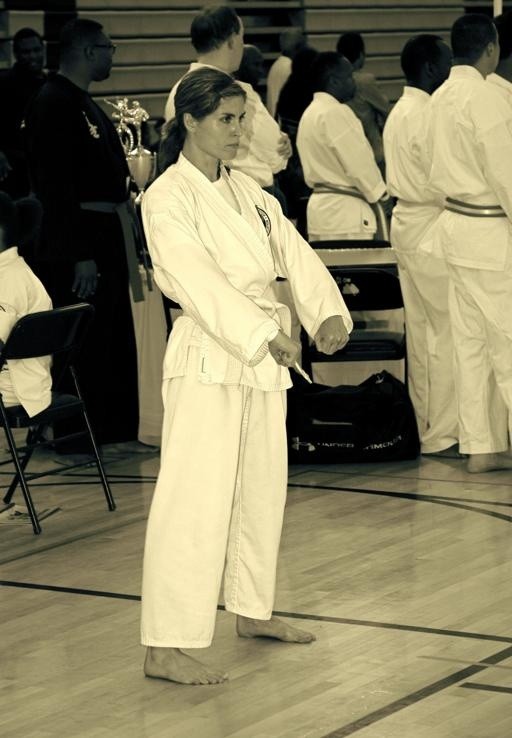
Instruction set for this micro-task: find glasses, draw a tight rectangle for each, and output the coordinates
[83,42,116,54]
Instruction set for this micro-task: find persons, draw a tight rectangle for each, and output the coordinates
[0,224,52,519]
[0,27,48,193]
[382,34,468,458]
[33,19,160,469]
[485,11,512,97]
[164,4,294,189]
[141,67,355,686]
[428,12,512,473]
[242,33,389,239]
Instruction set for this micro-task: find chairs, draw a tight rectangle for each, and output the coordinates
[0,303,116,534]
[299,267,407,448]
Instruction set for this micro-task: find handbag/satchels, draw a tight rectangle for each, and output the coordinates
[286,370,421,467]
